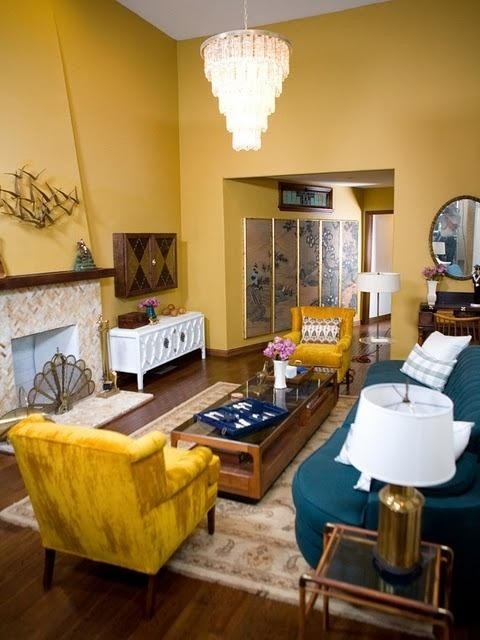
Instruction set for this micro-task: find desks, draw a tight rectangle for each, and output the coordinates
[417,291,480,347]
[298,522,454,639]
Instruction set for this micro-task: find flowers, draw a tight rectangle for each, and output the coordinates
[136,296,160,310]
[263,336,296,361]
[422,265,446,281]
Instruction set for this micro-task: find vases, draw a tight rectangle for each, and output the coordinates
[427,280,439,307]
[272,388,288,411]
[273,360,289,390]
[146,306,155,316]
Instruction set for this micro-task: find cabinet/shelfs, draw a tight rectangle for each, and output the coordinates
[108,310,206,391]
[278,181,334,212]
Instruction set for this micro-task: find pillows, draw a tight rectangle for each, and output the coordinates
[400,343,458,394]
[333,417,476,468]
[300,316,342,344]
[421,330,472,362]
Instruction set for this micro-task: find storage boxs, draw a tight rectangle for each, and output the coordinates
[117,310,148,328]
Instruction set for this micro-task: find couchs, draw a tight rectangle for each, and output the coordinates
[282,305,356,399]
[6,411,221,622]
[292,345,480,600]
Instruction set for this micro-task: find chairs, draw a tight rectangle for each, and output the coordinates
[433,313,480,345]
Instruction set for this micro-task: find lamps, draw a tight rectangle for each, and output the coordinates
[432,241,446,259]
[345,380,457,581]
[199,0,293,153]
[357,271,400,341]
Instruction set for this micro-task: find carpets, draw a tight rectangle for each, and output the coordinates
[0,388,154,454]
[0,380,434,637]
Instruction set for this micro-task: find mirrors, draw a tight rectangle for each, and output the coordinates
[429,195,480,281]
